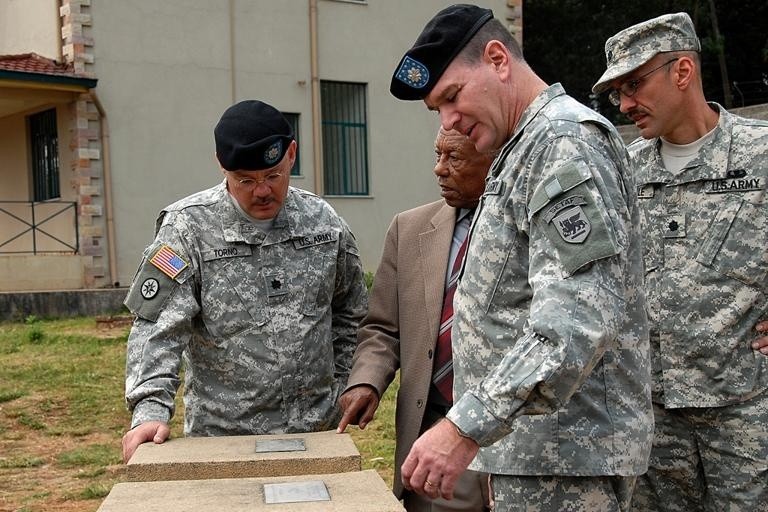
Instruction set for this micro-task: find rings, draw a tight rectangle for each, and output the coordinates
[425,478,436,487]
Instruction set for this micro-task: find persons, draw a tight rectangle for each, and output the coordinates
[120,101,367,463]
[592,12,768,511]
[388,5,654,511]
[337,120,504,511]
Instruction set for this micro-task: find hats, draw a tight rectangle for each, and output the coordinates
[214,99,296,172]
[592,11,702,91]
[390,3,494,101]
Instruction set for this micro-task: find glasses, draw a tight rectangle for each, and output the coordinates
[228,171,289,193]
[608,58,682,106]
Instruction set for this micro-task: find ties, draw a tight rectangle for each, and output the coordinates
[431,211,474,405]
